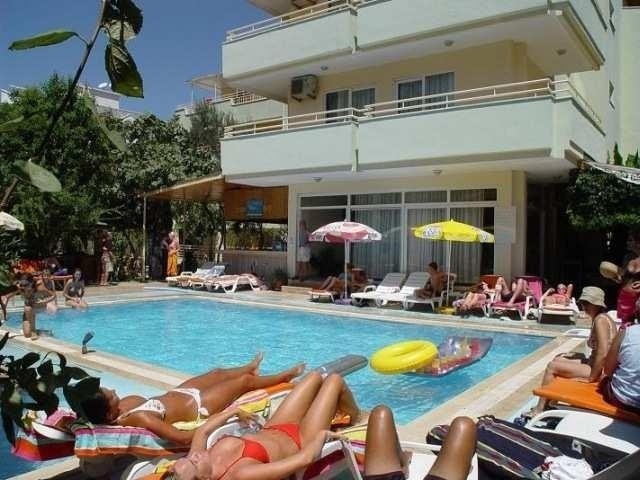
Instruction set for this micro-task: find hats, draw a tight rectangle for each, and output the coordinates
[577,287,608,309]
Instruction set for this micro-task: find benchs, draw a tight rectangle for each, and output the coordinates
[11,258,74,292]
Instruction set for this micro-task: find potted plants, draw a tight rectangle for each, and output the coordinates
[271,267,287,290]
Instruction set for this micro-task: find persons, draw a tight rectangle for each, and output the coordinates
[458,273,640,412]
[163,229,182,278]
[316,262,356,294]
[0,225,118,341]
[292,220,310,281]
[214,228,225,264]
[413,261,444,298]
[84,350,306,442]
[157,370,364,480]
[362,405,477,479]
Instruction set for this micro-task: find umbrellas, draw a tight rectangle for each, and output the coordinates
[0,211,24,233]
[417,218,494,312]
[309,216,385,306]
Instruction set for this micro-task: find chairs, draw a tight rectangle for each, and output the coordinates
[523,311,640,479]
[308,272,577,326]
[20,380,639,480]
[165,261,263,293]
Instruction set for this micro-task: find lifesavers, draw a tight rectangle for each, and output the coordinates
[370,340,437,375]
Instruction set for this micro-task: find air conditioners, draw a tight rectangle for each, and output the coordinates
[291,74,316,99]
